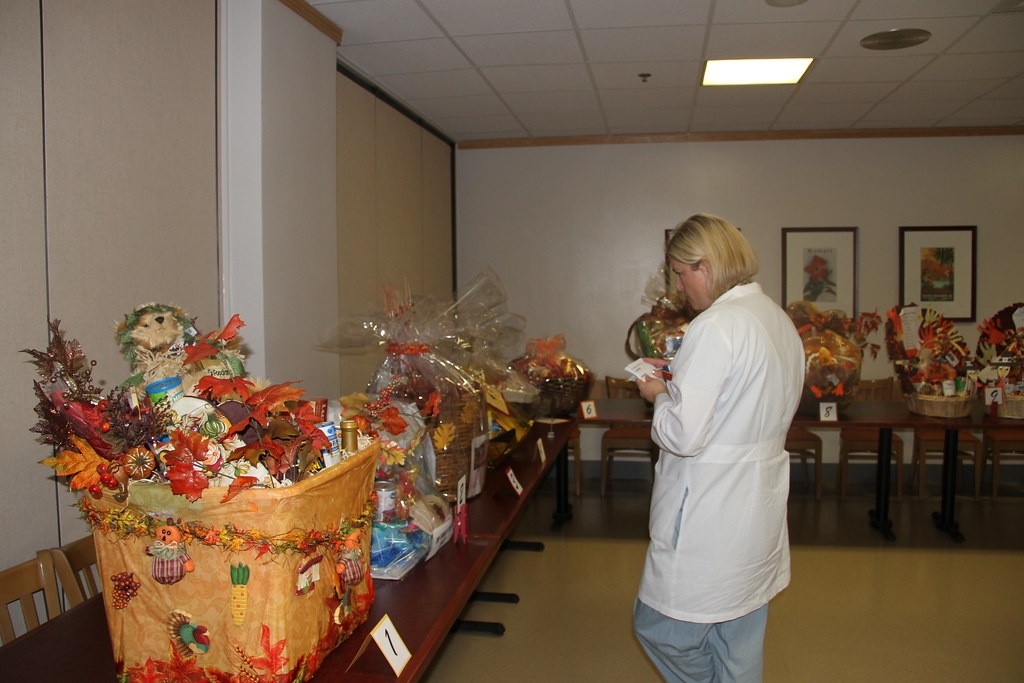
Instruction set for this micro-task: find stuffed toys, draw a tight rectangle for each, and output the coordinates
[335,527,369,585]
[140,513,195,586]
[109,300,247,419]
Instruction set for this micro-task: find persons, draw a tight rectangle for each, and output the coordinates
[623,211,810,683]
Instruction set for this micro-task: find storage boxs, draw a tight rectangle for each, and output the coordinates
[84,442,381,682]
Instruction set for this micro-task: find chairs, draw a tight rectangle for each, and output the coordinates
[51,535,103,608]
[0,548,61,646]
[837,377,904,502]
[600,375,658,497]
[567,428,581,495]
[982,429,1024,504]
[907,428,984,501]
[783,429,822,501]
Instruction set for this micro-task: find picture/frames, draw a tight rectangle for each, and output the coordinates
[781,226,856,323]
[898,225,977,322]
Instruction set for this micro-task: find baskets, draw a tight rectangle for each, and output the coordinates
[484,401,529,471]
[384,377,483,503]
[997,396,1024,420]
[904,392,978,417]
[796,392,854,416]
[506,362,592,416]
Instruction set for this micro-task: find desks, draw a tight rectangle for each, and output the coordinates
[0,406,582,683]
[581,399,1024,543]
